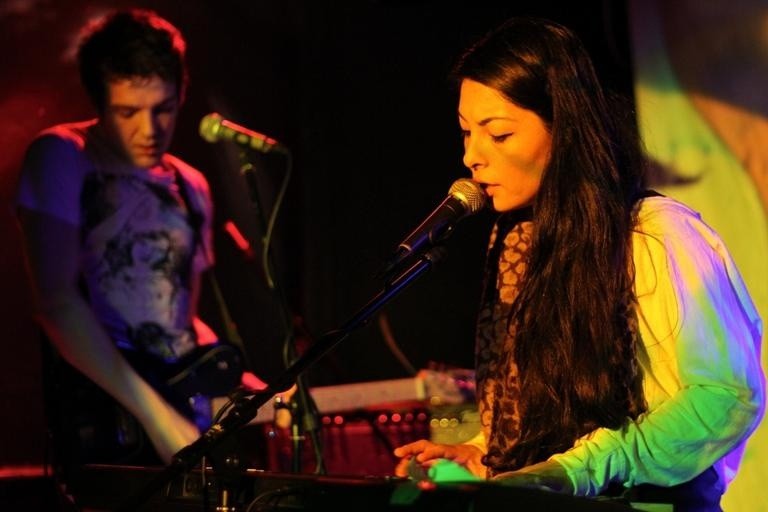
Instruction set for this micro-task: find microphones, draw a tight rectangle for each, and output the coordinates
[198,112,279,154]
[399,177,487,252]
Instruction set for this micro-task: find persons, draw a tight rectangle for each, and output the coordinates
[5,3,244,491]
[393,14,767,512]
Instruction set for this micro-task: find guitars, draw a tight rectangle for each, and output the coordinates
[68,332,332,512]
